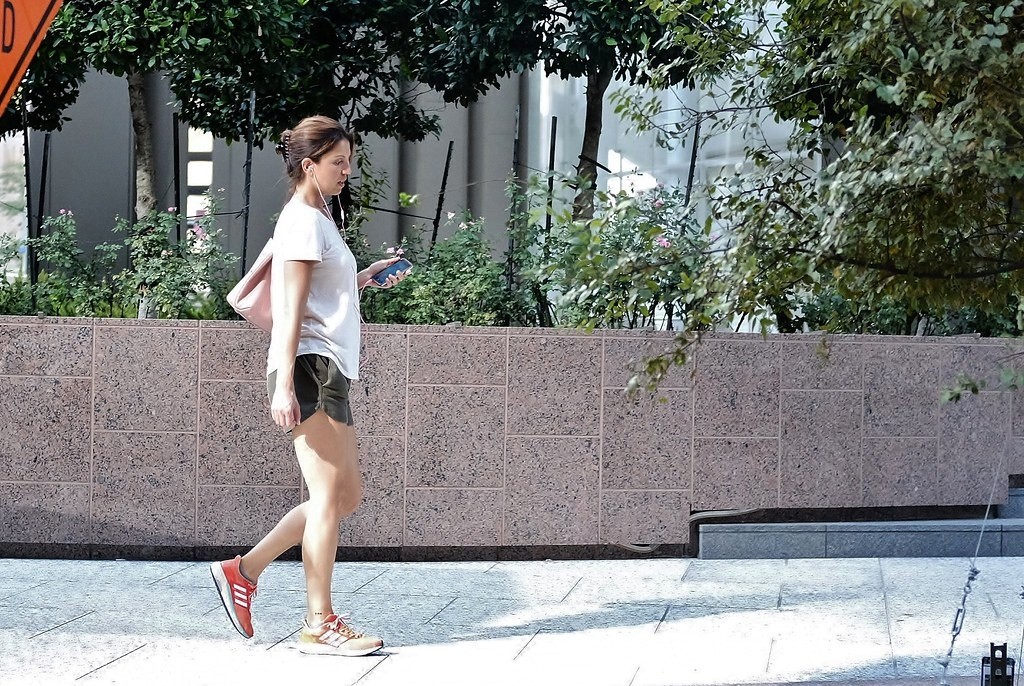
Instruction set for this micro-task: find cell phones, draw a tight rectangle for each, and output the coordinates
[370,257,413,286]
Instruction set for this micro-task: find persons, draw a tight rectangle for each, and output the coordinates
[208,115,411,657]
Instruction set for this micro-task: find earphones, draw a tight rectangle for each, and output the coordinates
[308,166,313,172]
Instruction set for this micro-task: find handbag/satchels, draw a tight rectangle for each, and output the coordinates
[225,237,274,333]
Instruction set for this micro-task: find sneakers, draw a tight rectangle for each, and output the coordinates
[210,555,258,639]
[299,614,383,657]
